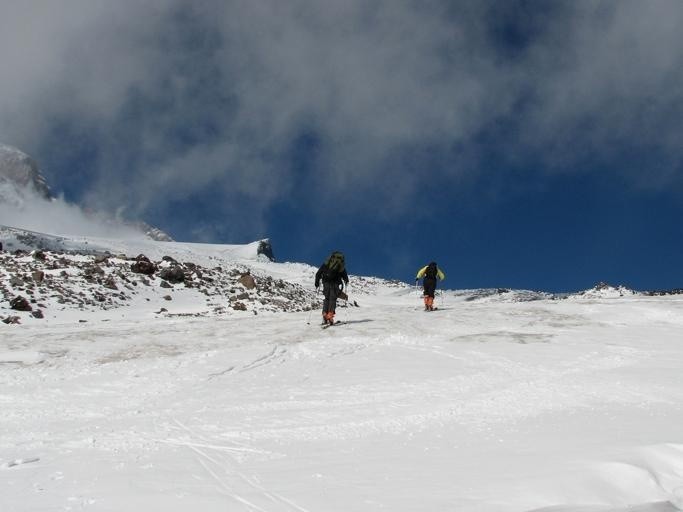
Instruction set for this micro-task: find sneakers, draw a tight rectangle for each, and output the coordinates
[323,312,327,324]
[327,311,334,325]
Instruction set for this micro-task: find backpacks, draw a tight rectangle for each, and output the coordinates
[328,253,344,274]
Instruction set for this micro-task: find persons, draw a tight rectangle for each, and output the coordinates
[415,262,445,311]
[314,251,348,325]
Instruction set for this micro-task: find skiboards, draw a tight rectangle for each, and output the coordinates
[423,308,438,313]
[320,320,341,332]
